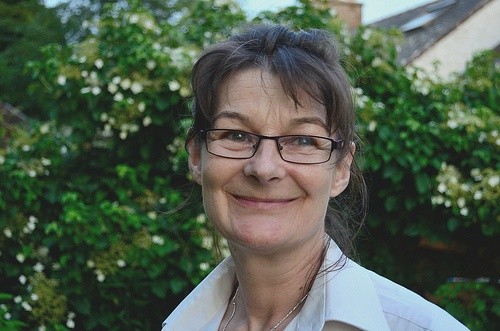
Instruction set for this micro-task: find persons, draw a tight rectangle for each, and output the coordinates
[159,25,471,331]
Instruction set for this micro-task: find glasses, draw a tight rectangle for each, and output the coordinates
[197,128,346,165]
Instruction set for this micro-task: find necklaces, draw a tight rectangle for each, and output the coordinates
[222,286,309,331]
